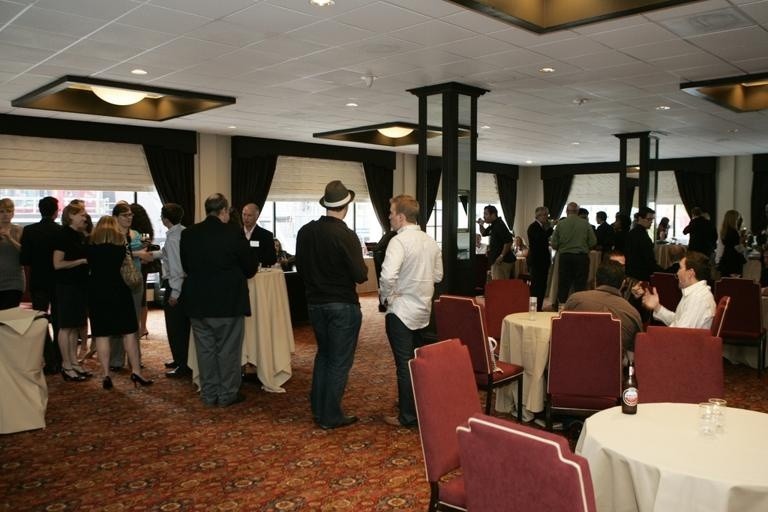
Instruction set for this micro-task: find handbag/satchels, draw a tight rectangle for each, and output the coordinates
[119,252,142,290]
[488,337,504,374]
[144,245,162,272]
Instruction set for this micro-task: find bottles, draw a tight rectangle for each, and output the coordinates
[528,296,537,321]
[620,360,639,414]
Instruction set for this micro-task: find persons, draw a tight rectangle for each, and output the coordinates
[140,191,297,409]
[0,192,155,390]
[477,203,768,440]
[296,180,368,429]
[376,195,444,427]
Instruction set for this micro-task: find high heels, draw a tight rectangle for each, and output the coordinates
[43,345,98,381]
[103,376,112,390]
[140,332,148,339]
[131,373,153,387]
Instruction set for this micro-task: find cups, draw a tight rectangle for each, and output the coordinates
[698,396,727,436]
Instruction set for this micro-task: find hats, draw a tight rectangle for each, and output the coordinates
[320,181,355,209]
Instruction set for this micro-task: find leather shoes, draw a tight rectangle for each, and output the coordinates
[314,416,357,429]
[215,393,244,407]
[165,361,192,378]
[383,416,403,427]
[109,364,144,371]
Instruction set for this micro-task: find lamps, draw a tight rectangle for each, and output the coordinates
[93,83,148,108]
[377,127,415,139]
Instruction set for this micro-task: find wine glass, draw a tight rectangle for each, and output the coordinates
[140,233,150,248]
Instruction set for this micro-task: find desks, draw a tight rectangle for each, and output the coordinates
[189,268,296,394]
[1,305,48,434]
